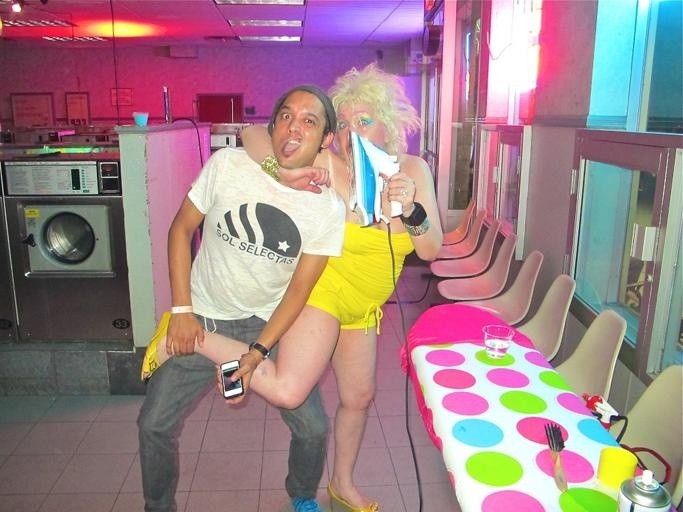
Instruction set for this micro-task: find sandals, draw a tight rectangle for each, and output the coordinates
[327,476,379,512]
[141,310,173,382]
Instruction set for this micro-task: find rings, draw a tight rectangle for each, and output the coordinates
[401,189,407,197]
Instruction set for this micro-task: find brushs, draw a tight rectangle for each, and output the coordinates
[544,422,567,491]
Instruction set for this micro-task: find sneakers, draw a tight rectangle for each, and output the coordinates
[289,497,321,512]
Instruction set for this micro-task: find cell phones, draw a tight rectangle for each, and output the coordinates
[220,359,244,398]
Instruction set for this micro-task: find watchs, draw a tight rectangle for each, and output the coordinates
[400,202,427,226]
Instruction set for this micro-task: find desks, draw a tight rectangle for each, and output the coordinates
[406,302,677,512]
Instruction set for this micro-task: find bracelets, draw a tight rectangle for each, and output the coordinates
[169,304,194,314]
[249,341,271,360]
[403,217,430,236]
[260,155,280,181]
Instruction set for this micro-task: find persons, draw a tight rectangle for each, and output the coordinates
[140,64,443,512]
[136,86,346,512]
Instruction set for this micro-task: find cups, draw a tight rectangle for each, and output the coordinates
[482,325,514,359]
[595,445,639,486]
[131,112,149,126]
